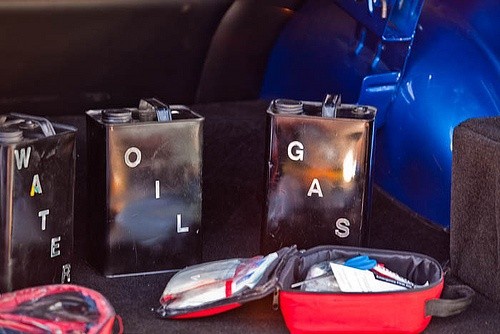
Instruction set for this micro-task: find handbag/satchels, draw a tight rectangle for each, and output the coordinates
[1,284,125,334]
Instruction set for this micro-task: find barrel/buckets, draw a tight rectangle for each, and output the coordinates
[0,112,79,293]
[85,100,205,278]
[261,99,378,256]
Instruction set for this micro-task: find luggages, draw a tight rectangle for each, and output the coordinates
[155,244,475,334]
